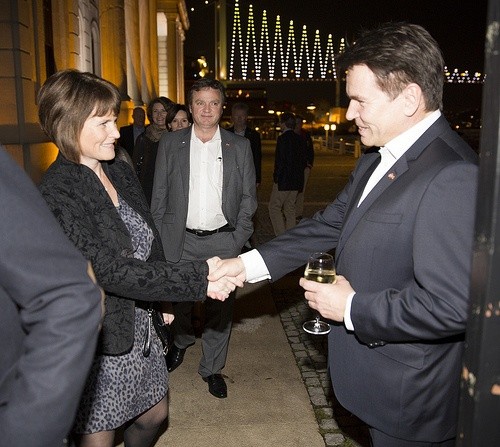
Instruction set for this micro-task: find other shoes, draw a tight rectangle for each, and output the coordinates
[295,215,301,222]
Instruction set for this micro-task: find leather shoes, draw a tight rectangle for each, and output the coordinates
[165,344,186,373]
[201,374,228,399]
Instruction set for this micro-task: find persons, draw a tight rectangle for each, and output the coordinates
[165,103,193,133]
[151,78,257,399]
[29,67,244,447]
[207,21,481,447]
[133,97,177,212]
[0,143,107,447]
[117,107,146,152]
[294,115,316,222]
[268,111,308,237]
[225,101,263,254]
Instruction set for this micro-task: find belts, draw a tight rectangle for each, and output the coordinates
[185,224,237,237]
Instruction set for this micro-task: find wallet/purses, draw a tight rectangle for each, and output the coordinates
[143,305,173,357]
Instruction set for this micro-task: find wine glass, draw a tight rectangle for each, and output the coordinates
[303,252,336,335]
